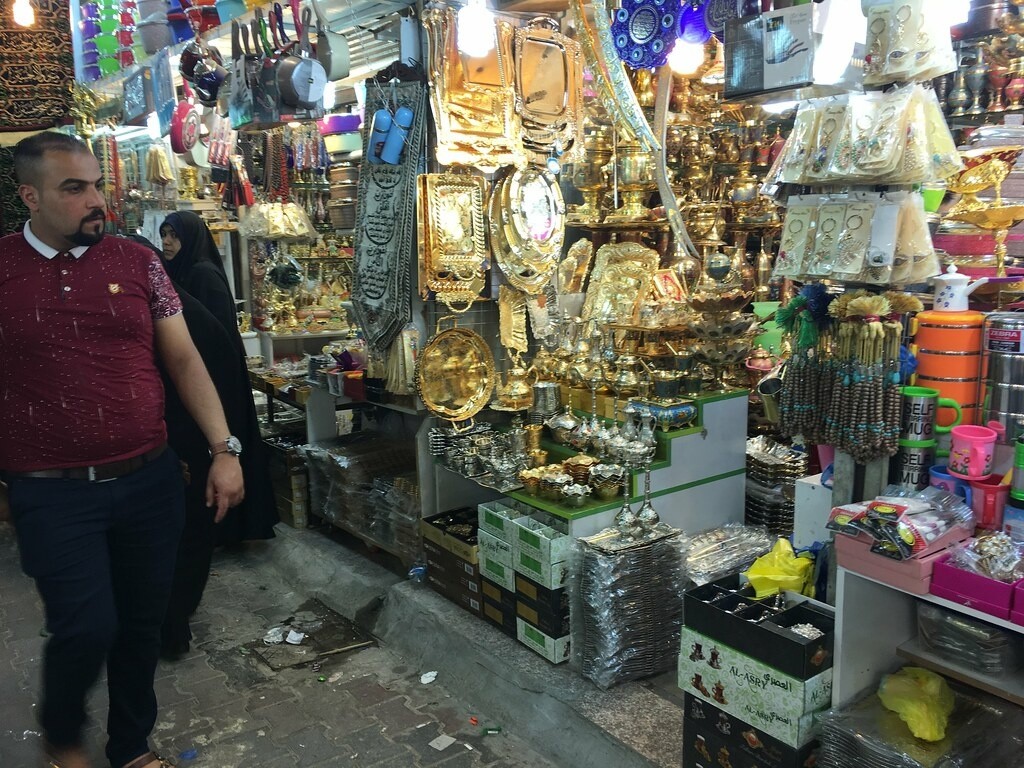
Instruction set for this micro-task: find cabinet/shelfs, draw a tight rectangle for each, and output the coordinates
[832,564,1024,768]
[311,379,751,666]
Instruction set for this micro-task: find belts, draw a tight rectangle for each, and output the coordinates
[9,441,167,480]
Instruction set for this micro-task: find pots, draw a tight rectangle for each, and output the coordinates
[415,316,495,434]
[171,4,350,152]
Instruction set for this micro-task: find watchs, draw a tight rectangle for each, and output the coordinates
[208,436,243,459]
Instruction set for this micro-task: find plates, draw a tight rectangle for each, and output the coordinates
[520,455,625,505]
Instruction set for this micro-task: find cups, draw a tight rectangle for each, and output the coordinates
[1010,440,1024,501]
[966,473,1009,531]
[898,438,952,494]
[949,425,996,482]
[929,466,973,513]
[900,387,963,440]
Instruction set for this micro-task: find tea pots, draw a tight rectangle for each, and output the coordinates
[929,265,989,310]
[496,365,539,410]
[552,346,651,398]
[531,345,552,381]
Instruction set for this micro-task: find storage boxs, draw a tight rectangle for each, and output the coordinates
[682,745,757,768]
[682,716,816,768]
[424,553,482,594]
[425,568,483,620]
[684,692,817,768]
[421,506,480,565]
[423,537,480,580]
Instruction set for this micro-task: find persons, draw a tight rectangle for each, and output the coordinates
[124,210,280,662]
[0,132,246,767]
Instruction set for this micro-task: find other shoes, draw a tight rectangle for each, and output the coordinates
[128,751,176,768]
[46,747,90,768]
